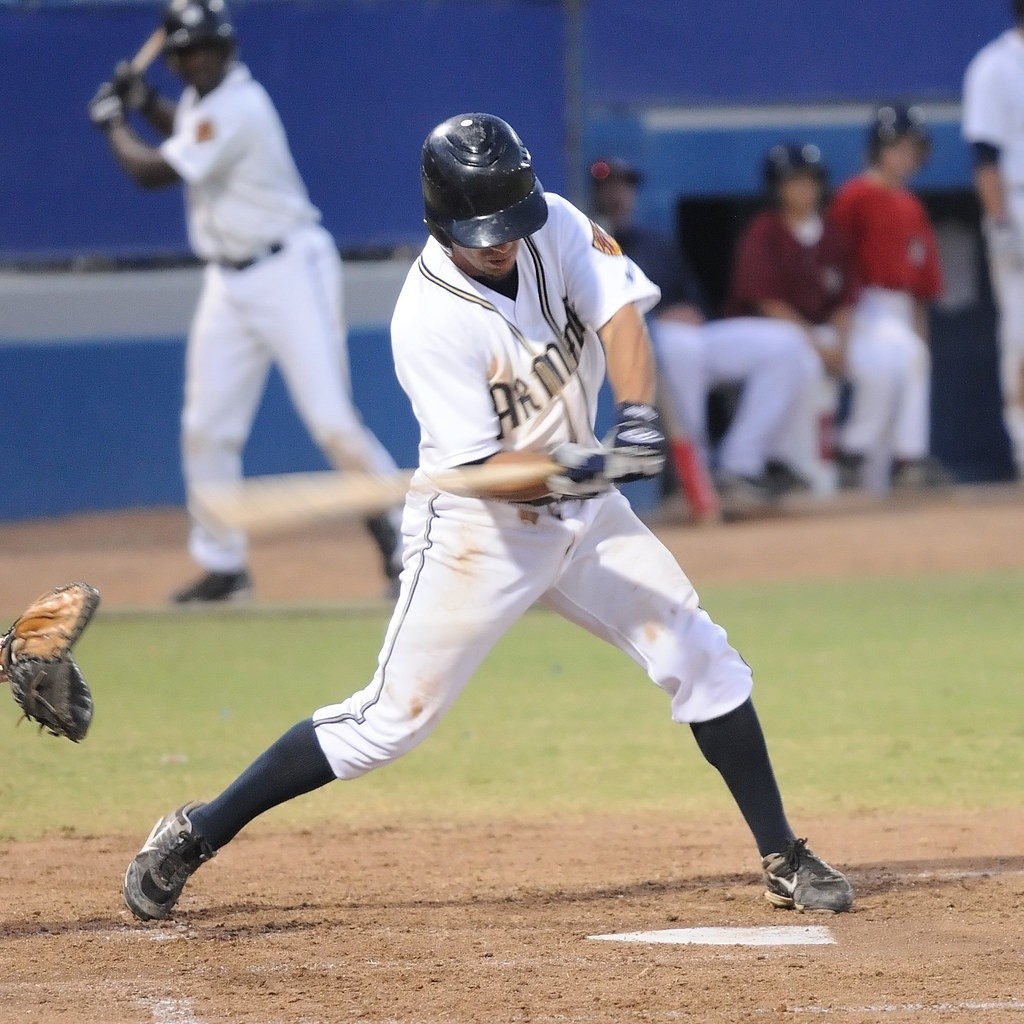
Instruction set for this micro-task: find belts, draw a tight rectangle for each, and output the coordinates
[216,244,281,271]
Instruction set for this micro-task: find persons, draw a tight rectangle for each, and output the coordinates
[92,0,406,602]
[123,114,853,921]
[590,0,1024,524]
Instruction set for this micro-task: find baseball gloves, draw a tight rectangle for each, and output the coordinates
[0,583,99,742]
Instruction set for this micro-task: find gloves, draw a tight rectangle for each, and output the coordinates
[545,402,666,498]
[87,59,156,131]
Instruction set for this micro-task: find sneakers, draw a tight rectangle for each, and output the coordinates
[830,448,865,487]
[174,570,251,604]
[761,838,852,911]
[722,459,809,503]
[365,502,404,580]
[890,456,971,489]
[123,801,218,922]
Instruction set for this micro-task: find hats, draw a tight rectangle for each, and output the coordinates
[759,146,824,184]
[587,156,641,186]
[864,107,932,156]
[161,0,235,51]
[422,111,549,250]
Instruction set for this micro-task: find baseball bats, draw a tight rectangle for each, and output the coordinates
[107,18,173,108]
[658,393,720,527]
[188,460,571,540]
